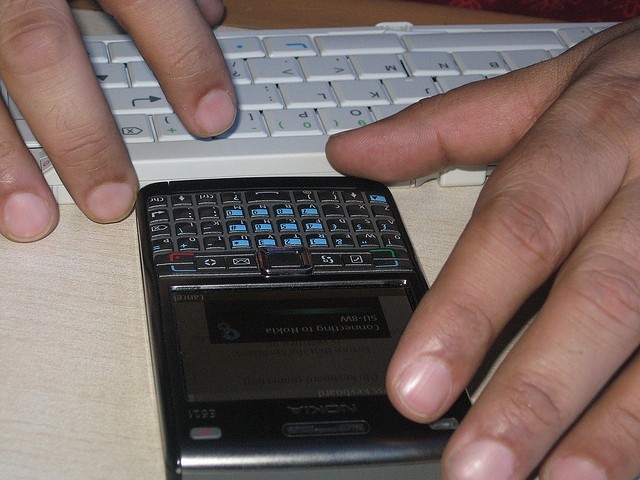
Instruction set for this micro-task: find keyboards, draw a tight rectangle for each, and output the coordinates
[1,19,628,205]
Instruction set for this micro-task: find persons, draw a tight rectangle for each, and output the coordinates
[0,0,640,478]
